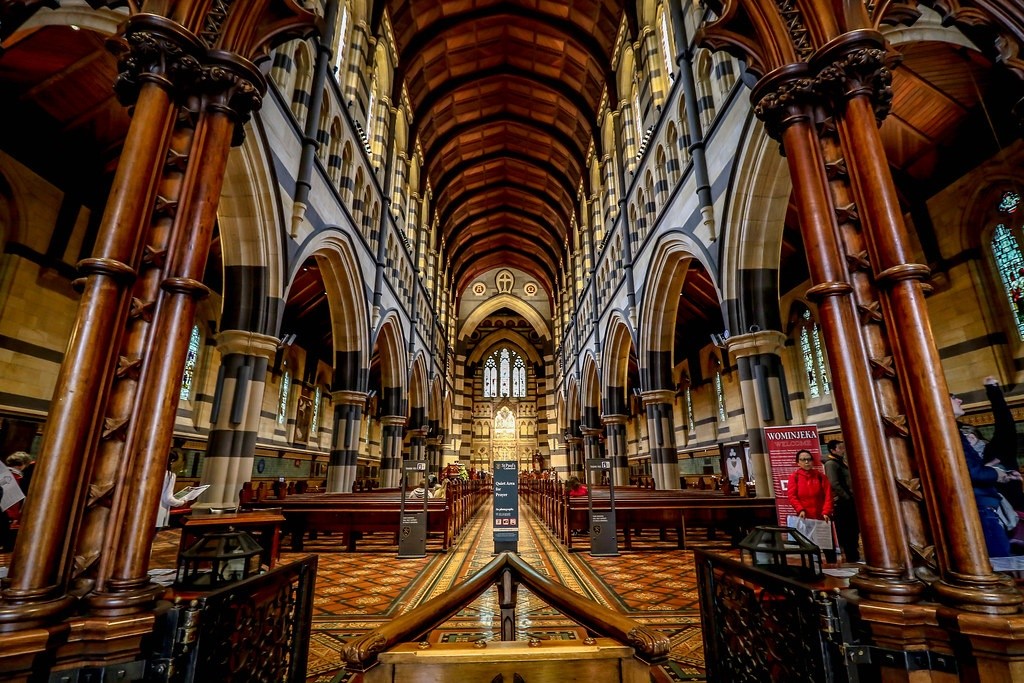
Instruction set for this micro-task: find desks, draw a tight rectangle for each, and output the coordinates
[173,510,286,591]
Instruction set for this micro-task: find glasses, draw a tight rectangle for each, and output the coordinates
[799,458,813,462]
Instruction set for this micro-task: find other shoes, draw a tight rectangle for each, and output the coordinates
[571,532,578,536]
[856,558,867,564]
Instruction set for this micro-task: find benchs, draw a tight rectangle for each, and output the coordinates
[520,476,776,552]
[239,479,491,553]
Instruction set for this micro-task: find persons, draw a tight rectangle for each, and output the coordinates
[1,452,187,534]
[533,459,542,471]
[786,440,860,564]
[409,468,588,538]
[949,393,1024,557]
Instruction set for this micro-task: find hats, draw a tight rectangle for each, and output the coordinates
[6,452,32,467]
